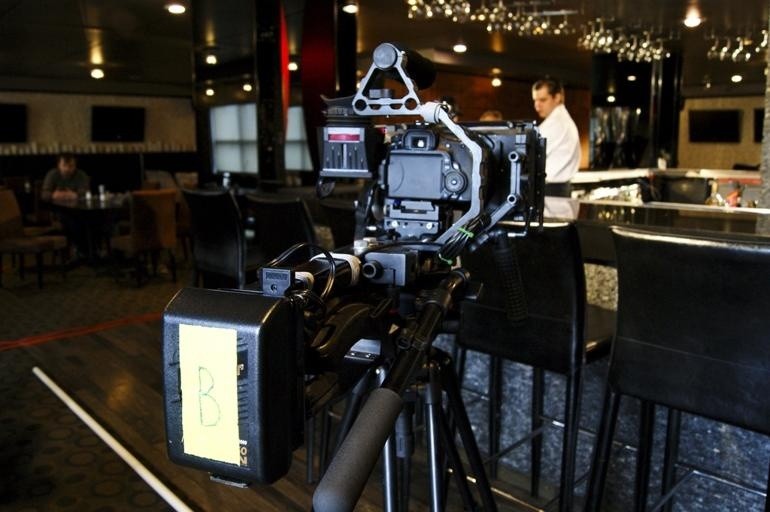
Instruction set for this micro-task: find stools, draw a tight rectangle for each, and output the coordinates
[444,222,655,511]
[581,224,770,510]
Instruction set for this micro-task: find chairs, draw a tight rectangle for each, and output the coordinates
[2,176,769,290]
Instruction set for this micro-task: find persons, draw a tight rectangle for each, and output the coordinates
[41,154,98,260]
[533,80,582,219]
[480,110,503,122]
[441,96,459,122]
